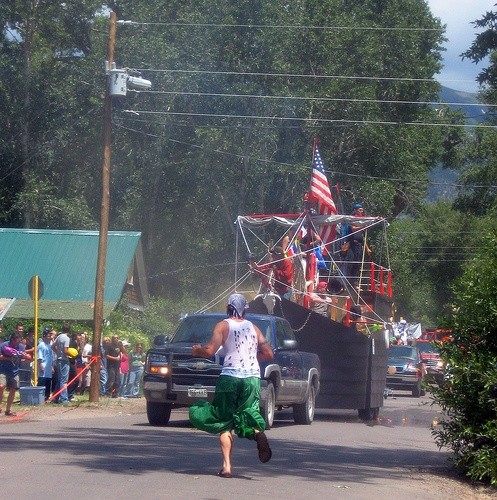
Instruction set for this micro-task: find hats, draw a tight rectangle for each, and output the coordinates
[122,341,131,346]
[316,282,327,291]
[353,204,362,210]
[326,279,344,294]
[269,246,285,255]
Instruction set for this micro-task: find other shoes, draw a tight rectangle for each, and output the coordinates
[5,410,16,416]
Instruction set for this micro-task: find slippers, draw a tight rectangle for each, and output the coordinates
[218,470,231,478]
[254,431,270,463]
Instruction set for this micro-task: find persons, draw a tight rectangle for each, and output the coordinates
[307,224,327,272]
[51,322,78,405]
[249,246,294,302]
[69,332,132,398]
[310,282,332,318]
[125,343,146,398]
[0,333,26,416]
[386,320,420,345]
[37,330,55,401]
[23,327,38,357]
[341,204,371,294]
[38,327,54,346]
[16,325,24,334]
[190,294,273,478]
[341,305,362,330]
[282,229,297,254]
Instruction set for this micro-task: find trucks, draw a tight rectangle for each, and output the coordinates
[405,339,448,389]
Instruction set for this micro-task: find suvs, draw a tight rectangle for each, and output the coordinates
[382,346,428,399]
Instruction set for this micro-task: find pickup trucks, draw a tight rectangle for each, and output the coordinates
[142,314,322,431]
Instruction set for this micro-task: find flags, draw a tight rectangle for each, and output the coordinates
[310,144,337,255]
